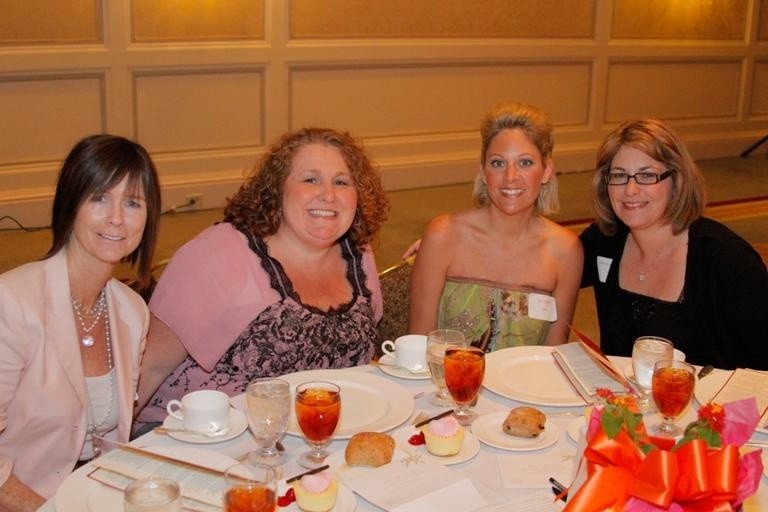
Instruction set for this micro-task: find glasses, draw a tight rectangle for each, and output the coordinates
[605,170,674,185]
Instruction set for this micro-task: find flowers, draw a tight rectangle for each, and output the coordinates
[598,389,726,454]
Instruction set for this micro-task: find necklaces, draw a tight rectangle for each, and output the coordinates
[71,287,114,452]
[639,236,684,282]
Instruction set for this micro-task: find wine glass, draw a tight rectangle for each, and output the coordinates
[443,346,487,422]
[426,332,464,408]
[649,359,694,437]
[632,336,674,415]
[294,382,340,469]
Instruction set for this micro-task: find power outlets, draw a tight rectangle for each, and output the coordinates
[185,194,202,208]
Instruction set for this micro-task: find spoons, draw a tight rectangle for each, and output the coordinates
[369,359,426,374]
[154,427,223,439]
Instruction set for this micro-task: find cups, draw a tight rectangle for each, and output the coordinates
[221,466,279,511]
[245,380,291,467]
[124,476,184,511]
[377,351,434,380]
[381,335,432,367]
[166,389,233,435]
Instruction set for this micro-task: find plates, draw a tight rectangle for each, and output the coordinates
[324,445,409,483]
[389,424,480,465]
[481,346,591,406]
[163,406,248,443]
[268,477,356,511]
[470,410,561,451]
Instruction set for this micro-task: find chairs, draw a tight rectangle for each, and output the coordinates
[128,261,170,302]
[378,253,414,356]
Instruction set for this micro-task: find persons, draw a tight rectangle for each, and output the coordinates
[129,127,391,440]
[1,133,162,512]
[402,117,768,370]
[408,102,585,346]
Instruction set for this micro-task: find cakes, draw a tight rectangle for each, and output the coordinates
[503,406,546,437]
[292,474,340,511]
[345,432,396,468]
[421,416,464,457]
